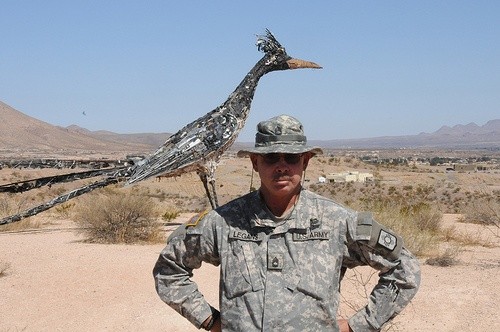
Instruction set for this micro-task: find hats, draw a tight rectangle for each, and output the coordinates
[235,114,323,158]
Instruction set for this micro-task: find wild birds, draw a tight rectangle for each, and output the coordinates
[0,25,324,225]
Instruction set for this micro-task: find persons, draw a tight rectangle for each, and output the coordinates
[152,114,424,332]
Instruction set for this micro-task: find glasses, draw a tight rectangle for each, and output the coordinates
[259,153,304,163]
